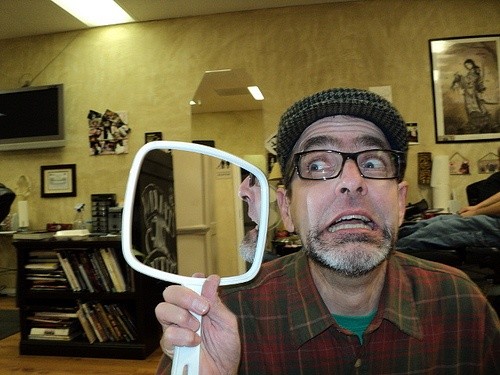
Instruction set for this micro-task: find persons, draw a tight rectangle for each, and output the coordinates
[154,89,500,375]
[238,173,261,264]
[393,190,500,253]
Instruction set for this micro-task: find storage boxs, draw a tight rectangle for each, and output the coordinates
[90,194,123,234]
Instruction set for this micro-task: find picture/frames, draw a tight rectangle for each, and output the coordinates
[40,164,77,197]
[428,34,500,144]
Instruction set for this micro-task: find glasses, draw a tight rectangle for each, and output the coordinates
[285,148,406,188]
[249,171,256,188]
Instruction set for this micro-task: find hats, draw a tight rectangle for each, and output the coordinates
[276,87,407,176]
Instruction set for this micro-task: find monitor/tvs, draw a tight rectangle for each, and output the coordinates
[0,83,66,151]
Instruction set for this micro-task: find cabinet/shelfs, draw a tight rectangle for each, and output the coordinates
[13,232,154,360]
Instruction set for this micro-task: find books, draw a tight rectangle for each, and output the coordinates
[23,246,139,345]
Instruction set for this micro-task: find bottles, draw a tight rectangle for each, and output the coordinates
[72,209,85,229]
